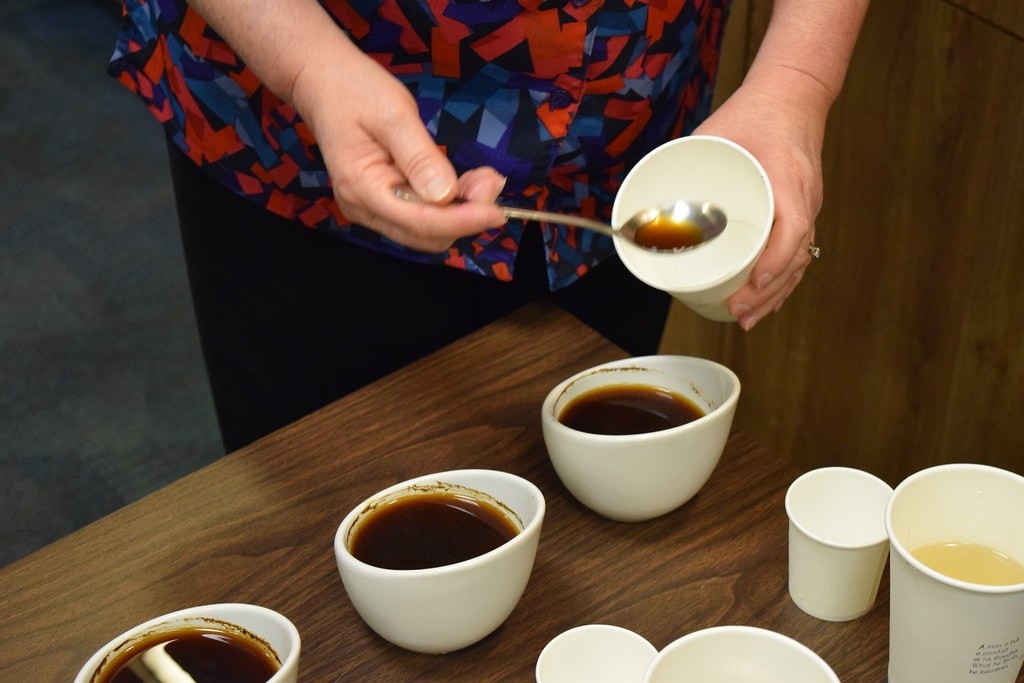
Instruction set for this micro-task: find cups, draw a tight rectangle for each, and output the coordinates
[644,626,840,683]
[610,137,774,324]
[885,463,1024,683]
[535,624,658,683]
[783,467,893,621]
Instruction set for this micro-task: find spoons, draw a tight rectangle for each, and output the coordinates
[389,182,726,250]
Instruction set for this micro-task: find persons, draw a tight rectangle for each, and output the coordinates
[103,0,876,454]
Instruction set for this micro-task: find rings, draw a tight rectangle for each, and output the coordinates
[808,242,821,259]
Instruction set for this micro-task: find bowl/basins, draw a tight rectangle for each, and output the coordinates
[73,602,301,683]
[541,352,741,523]
[334,469,544,654]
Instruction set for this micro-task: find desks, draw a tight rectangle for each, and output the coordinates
[0,298,1024,683]
